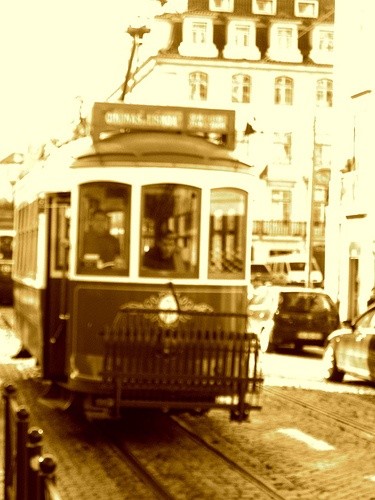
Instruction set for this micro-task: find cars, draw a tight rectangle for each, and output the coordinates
[246,239,326,294]
[269,288,340,353]
[322,304,375,383]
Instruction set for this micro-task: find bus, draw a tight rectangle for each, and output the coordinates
[0,101,266,427]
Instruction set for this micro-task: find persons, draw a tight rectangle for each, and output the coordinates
[82,209,121,262]
[138,228,194,272]
